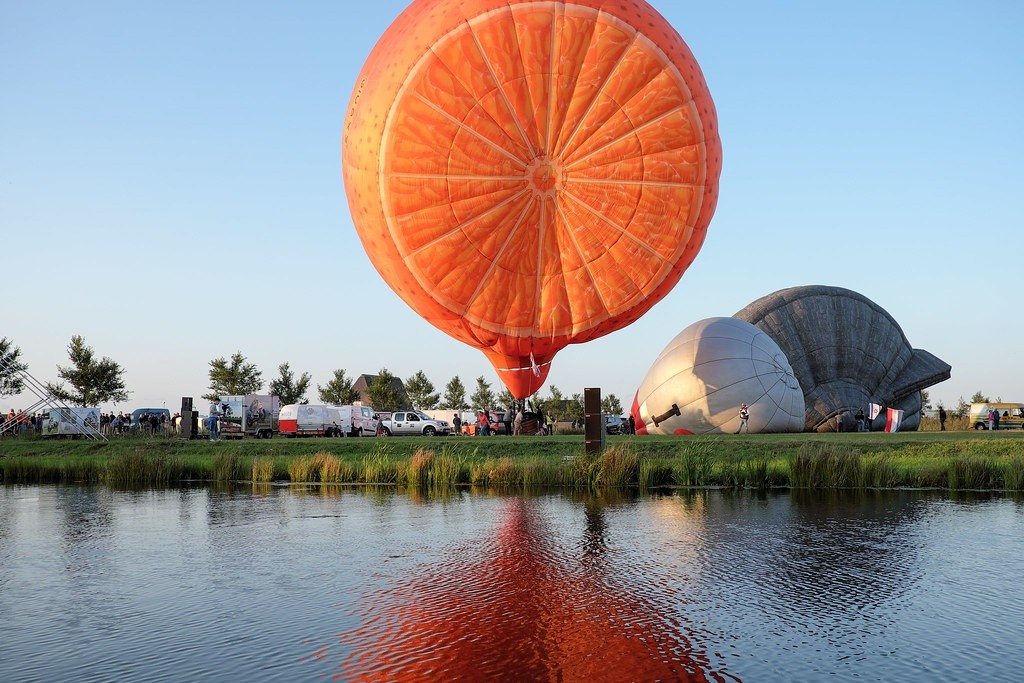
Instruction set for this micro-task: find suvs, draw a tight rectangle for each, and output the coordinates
[120,408,170,432]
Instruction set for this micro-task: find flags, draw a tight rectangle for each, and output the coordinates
[884,408,904,432]
[868,403,882,420]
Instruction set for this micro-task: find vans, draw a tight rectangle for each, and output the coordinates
[279,404,380,439]
[970,401,1024,430]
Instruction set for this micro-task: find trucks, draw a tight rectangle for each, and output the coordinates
[218,395,284,439]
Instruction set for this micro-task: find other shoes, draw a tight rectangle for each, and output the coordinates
[216,439,222,441]
[210,439,215,442]
[734,432,739,434]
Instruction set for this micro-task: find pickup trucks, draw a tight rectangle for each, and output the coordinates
[381,410,451,437]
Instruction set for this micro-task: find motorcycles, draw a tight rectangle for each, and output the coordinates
[247,405,266,428]
[84,416,99,430]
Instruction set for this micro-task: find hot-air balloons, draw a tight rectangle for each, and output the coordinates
[619,317,807,436]
[730,284,952,435]
[341,0,723,435]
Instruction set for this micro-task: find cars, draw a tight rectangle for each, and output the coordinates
[461,411,516,436]
[605,416,623,435]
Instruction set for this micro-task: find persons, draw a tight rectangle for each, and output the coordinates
[100,411,131,434]
[857,407,865,432]
[733,403,750,436]
[835,412,844,433]
[208,398,223,442]
[377,420,384,436]
[0,409,42,433]
[138,412,181,435]
[939,406,947,431]
[987,408,1000,430]
[453,406,554,437]
[629,416,635,435]
[572,417,583,428]
